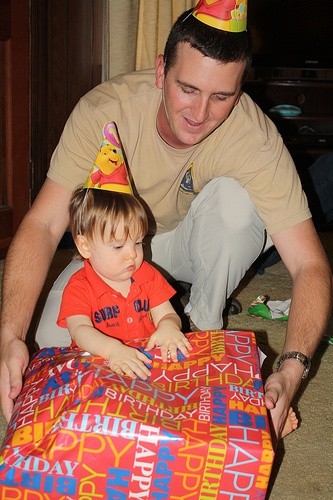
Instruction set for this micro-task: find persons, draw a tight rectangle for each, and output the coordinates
[0,0,333,452]
[57,120,299,440]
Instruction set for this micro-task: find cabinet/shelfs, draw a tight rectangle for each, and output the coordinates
[239,59,333,205]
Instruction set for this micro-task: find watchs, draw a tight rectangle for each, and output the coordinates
[277,351,312,381]
